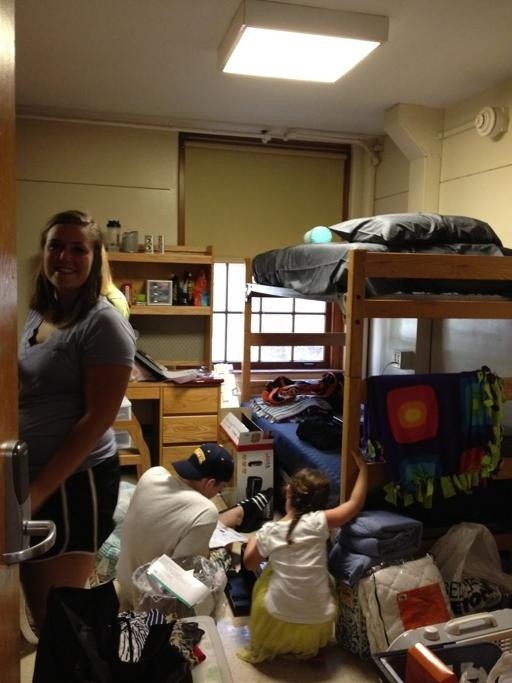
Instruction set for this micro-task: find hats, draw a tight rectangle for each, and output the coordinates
[172,443,234,482]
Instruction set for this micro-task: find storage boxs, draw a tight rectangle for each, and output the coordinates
[218,409,276,520]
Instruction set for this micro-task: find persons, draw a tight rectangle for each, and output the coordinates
[115,442,270,605]
[235,447,370,664]
[101,242,131,321]
[16,211,135,637]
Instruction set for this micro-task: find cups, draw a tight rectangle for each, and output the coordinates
[106,220,121,252]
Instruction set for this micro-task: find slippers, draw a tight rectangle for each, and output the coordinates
[219,486,274,532]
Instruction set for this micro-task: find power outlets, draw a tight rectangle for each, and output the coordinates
[392,349,411,369]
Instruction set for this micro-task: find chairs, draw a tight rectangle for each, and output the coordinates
[109,412,152,482]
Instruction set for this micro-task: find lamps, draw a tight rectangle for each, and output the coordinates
[218,1,389,84]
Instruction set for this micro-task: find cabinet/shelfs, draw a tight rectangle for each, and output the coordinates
[100,243,213,367]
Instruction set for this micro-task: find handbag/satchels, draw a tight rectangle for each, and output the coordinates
[297,422,341,451]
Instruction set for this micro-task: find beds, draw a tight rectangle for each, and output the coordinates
[240,242,512,561]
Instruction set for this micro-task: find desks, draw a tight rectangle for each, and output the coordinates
[123,378,224,477]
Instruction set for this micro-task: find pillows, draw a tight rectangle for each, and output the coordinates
[328,212,504,248]
